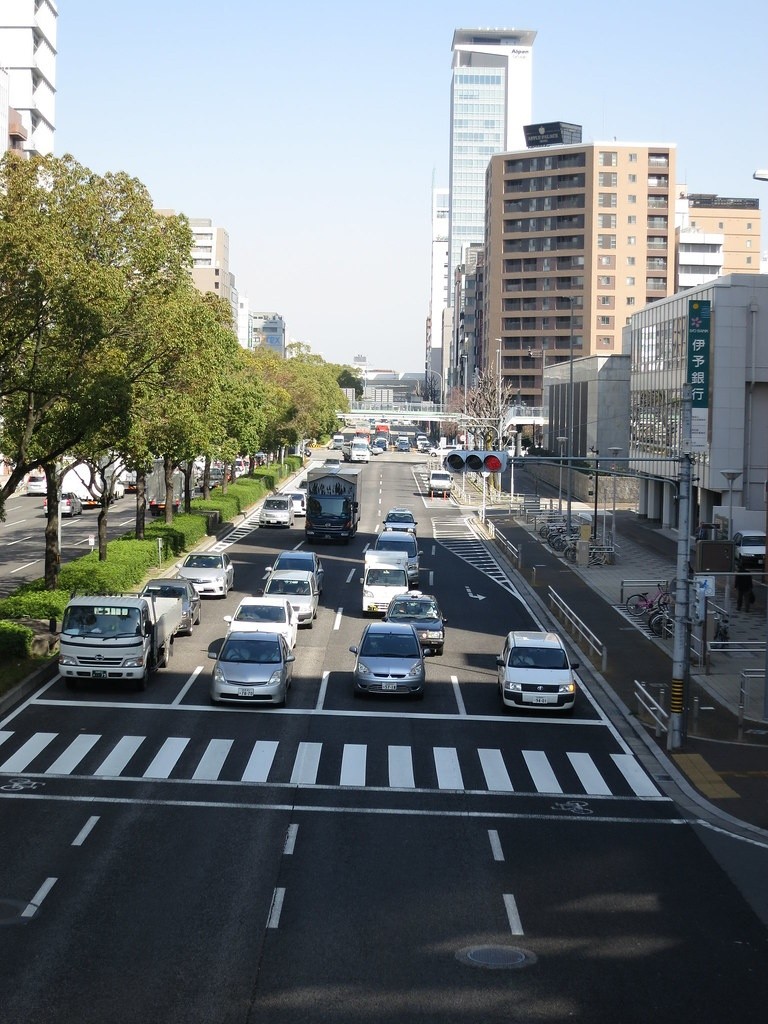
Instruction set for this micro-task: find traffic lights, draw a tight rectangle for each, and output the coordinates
[445,450,508,473]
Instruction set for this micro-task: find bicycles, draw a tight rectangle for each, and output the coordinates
[714,609,729,648]
[626,581,674,637]
[539,518,607,565]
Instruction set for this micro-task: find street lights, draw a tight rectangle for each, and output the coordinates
[720,470,743,541]
[607,447,622,543]
[556,436,568,514]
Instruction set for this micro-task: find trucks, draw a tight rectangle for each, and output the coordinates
[49,591,182,690]
[301,467,362,546]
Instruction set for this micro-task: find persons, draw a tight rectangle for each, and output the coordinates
[734,565,753,613]
[694,522,709,541]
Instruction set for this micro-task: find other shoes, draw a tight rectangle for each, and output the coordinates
[736,607,741,611]
[745,610,749,613]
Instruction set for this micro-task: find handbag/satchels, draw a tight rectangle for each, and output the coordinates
[750,592,756,604]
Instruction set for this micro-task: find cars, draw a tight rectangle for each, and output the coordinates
[349,623,431,700]
[322,421,454,497]
[206,631,295,707]
[360,507,424,616]
[223,551,324,650]
[257,479,309,528]
[25,451,273,516]
[137,552,233,636]
[732,530,766,568]
[381,590,447,656]
[496,631,579,709]
